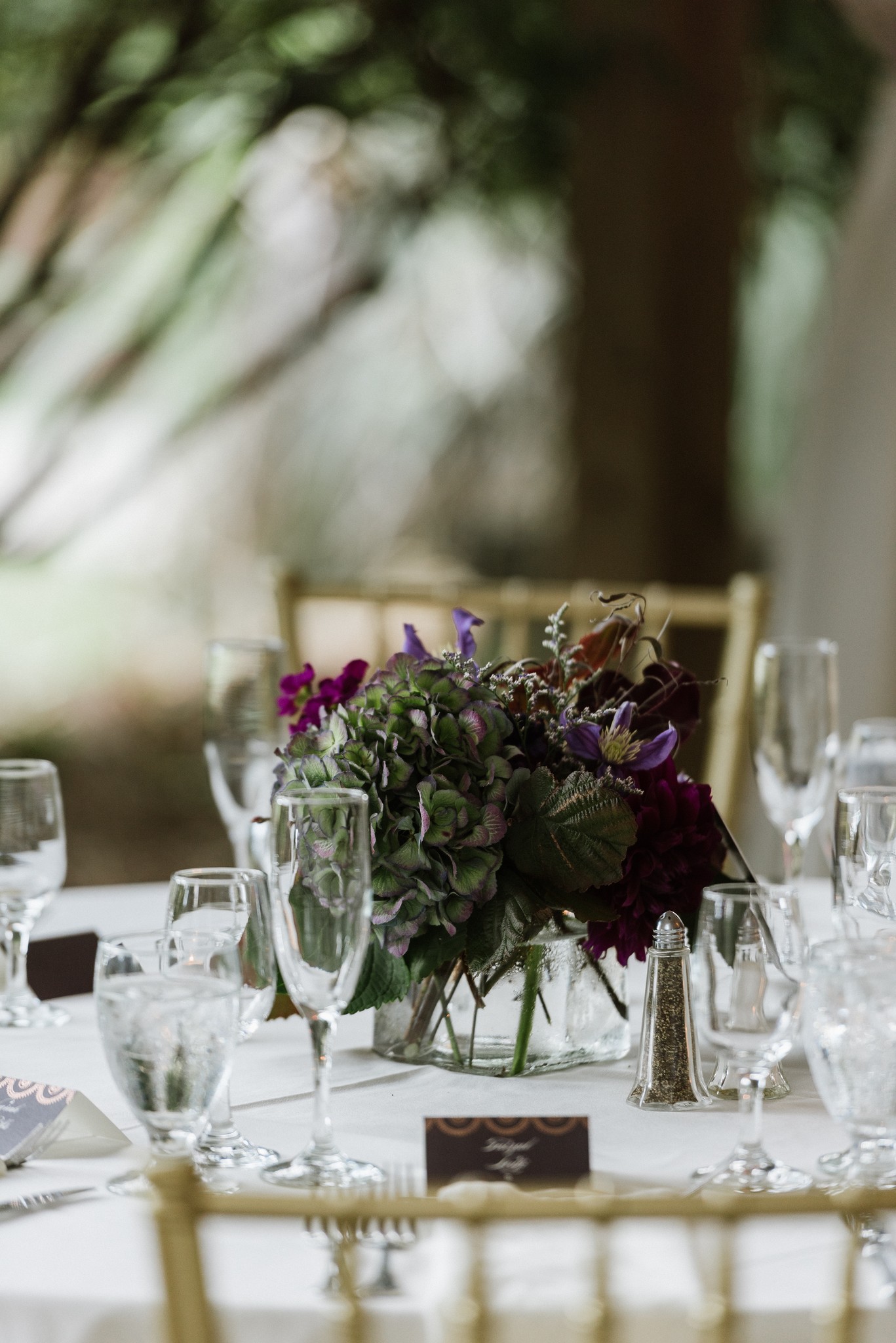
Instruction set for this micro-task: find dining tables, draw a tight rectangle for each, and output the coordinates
[0,878,896,1343]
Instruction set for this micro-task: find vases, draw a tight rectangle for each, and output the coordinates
[366,908,648,1071]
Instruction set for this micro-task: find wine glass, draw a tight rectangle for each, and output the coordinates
[695,882,815,1196]
[159,869,278,1170]
[751,639,841,965]
[261,788,388,1188]
[0,758,69,1025]
[93,931,238,1198]
[202,634,290,868]
[800,935,893,1186]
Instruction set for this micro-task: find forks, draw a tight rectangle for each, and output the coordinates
[1,1119,66,1177]
[305,1177,362,1296]
[367,1161,428,1297]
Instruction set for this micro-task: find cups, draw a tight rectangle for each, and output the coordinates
[831,784,896,938]
[826,720,893,790]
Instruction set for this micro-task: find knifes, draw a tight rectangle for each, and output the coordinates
[0,1186,90,1213]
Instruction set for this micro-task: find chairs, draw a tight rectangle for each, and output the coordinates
[271,564,770,876]
[149,1155,896,1341]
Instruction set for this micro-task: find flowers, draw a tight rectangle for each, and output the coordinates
[261,589,761,1071]
